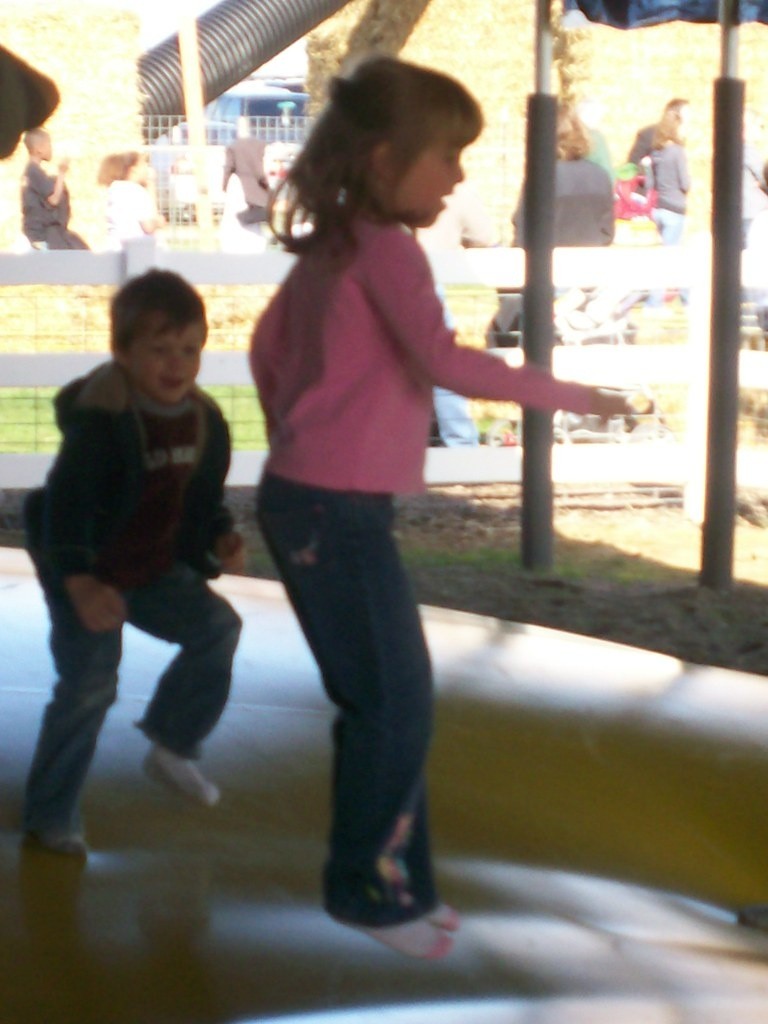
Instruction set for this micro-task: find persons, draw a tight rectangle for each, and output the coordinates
[250,56,656,960]
[627,98,698,347]
[20,129,91,250]
[484,92,616,348]
[97,150,166,251]
[223,116,479,448]
[22,266,246,857]
